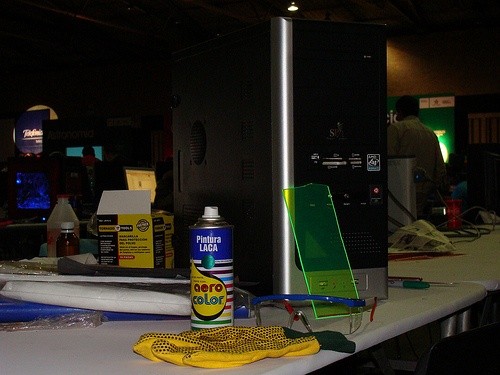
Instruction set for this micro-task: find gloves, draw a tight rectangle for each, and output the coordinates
[132,325,356,369]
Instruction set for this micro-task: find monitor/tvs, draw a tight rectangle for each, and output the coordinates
[65,146,104,162]
[122,166,159,205]
[4,161,59,223]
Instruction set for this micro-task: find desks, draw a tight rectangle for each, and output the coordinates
[388,224,500,332]
[0,284,487,375]
[0,220,88,261]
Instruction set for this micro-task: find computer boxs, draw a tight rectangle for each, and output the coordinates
[169,16,388,307]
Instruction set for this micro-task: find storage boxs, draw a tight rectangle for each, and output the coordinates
[96,189,175,269]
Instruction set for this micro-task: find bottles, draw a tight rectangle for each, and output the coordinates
[47,194,80,258]
[55,221,80,258]
[189,207,235,330]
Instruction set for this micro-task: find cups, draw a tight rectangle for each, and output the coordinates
[446,200,463,228]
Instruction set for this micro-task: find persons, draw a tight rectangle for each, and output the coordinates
[69,144,127,205]
[388,96,446,221]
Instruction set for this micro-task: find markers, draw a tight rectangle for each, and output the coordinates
[387,278,430,289]
